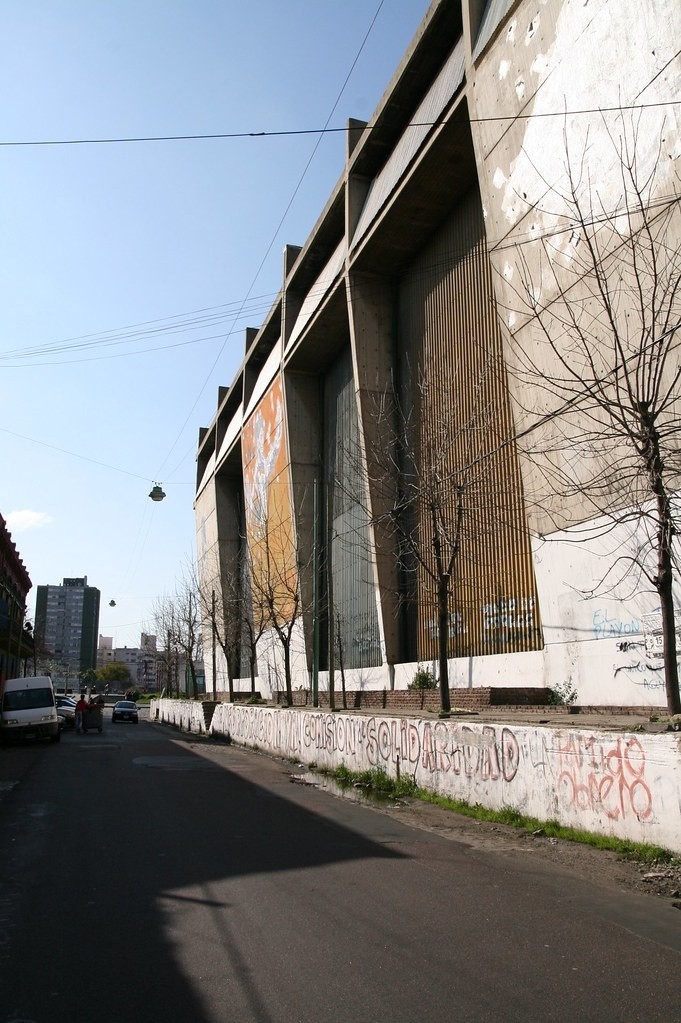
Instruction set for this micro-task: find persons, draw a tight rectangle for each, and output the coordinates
[74,695,104,734]
[125,690,138,702]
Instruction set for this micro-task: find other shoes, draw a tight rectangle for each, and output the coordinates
[77,728,80,733]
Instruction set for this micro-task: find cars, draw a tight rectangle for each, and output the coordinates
[110,700,141,724]
[56,694,79,731]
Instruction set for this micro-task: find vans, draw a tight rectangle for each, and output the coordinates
[0,675,62,743]
